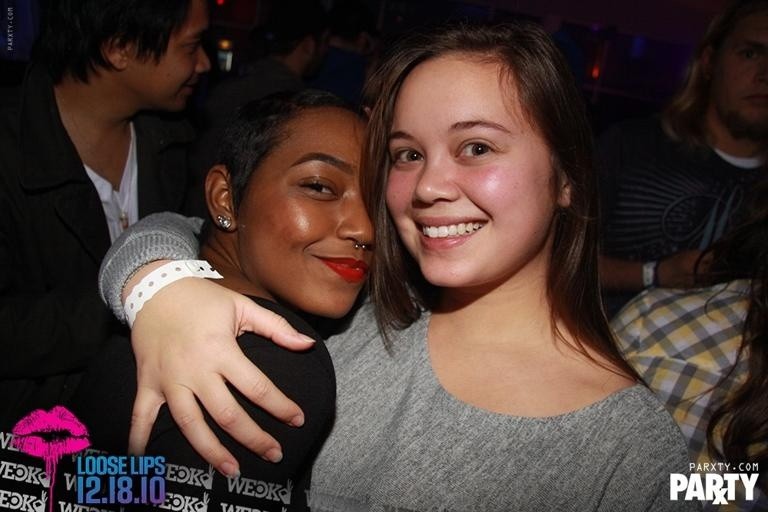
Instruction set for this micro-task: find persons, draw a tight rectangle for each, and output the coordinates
[595,0,767,320]
[193,0,700,135]
[0,1,212,453]
[93,16,699,512]
[0,88,374,512]
[605,173,766,511]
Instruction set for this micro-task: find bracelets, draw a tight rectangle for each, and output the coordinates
[111,261,221,323]
[641,258,657,286]
